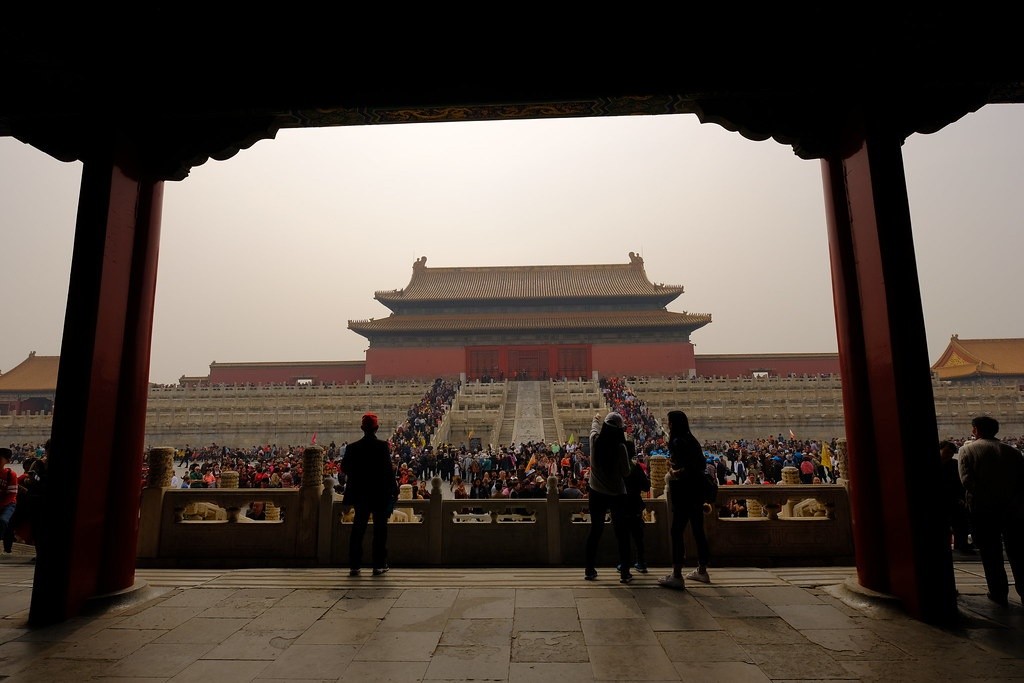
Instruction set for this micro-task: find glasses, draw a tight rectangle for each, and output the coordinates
[196,469,201,471]
[216,467,219,468]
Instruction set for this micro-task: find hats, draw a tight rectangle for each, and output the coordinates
[404,440,417,449]
[395,455,399,457]
[612,394,615,396]
[439,442,457,457]
[749,474,756,478]
[466,448,504,459]
[727,480,734,485]
[702,451,724,463]
[408,468,413,471]
[765,442,795,460]
[401,463,407,469]
[361,414,378,427]
[604,412,625,427]
[411,456,415,459]
[758,470,763,473]
[762,481,770,486]
[637,450,671,458]
[425,449,428,452]
[536,476,544,483]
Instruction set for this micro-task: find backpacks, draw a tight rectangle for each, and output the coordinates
[188,450,193,457]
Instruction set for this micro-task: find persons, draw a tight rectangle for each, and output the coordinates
[341,415,400,577]
[586,412,651,583]
[938,419,1024,608]
[0,368,1024,564]
[661,411,718,591]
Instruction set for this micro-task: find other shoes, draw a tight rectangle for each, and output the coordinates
[617,564,622,570]
[634,562,648,573]
[658,575,686,589]
[619,573,633,583]
[349,569,361,577]
[584,566,598,580]
[0,551,12,557]
[686,570,710,580]
[373,566,389,575]
